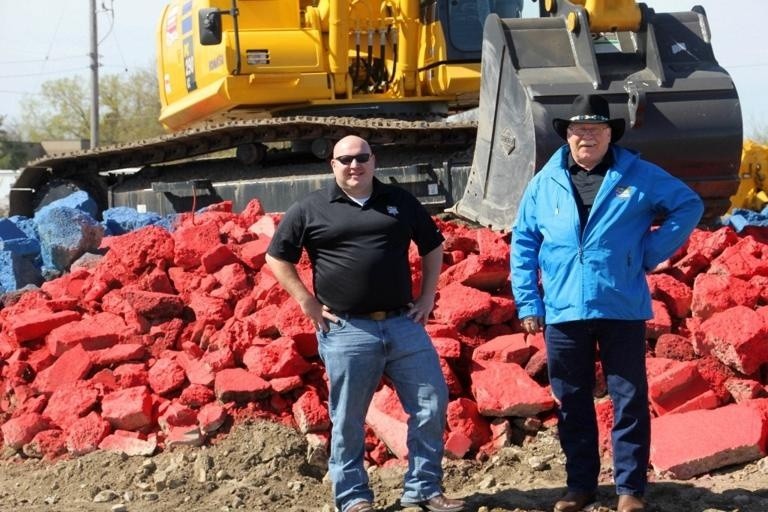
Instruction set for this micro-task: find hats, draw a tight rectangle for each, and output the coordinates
[552,92,628,144]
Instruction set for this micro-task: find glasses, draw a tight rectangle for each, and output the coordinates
[564,123,613,138]
[335,152,374,166]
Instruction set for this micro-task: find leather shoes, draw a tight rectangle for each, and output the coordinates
[398,492,469,512]
[615,492,649,511]
[336,499,374,512]
[551,488,601,512]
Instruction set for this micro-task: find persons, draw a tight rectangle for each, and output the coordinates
[265,135,467,512]
[509,95,705,512]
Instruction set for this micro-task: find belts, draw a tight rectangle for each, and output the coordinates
[322,304,417,323]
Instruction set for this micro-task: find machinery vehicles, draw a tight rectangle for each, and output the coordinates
[8,1,744,232]
